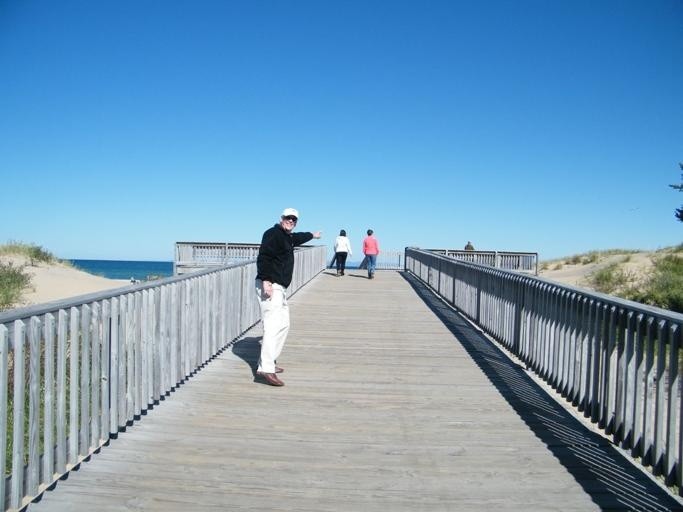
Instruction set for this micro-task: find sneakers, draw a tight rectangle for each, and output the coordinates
[368,272,375,279]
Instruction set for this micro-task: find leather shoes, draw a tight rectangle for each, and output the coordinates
[256,366,285,386]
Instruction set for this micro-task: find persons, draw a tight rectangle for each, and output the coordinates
[363,229,379,278]
[255,208,323,386]
[334,230,353,276]
[465,241,474,250]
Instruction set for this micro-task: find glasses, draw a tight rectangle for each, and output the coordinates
[286,216,296,221]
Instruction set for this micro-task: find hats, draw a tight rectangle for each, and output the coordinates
[281,208,298,219]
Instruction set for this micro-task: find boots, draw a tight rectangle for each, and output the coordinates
[336,268,345,277]
